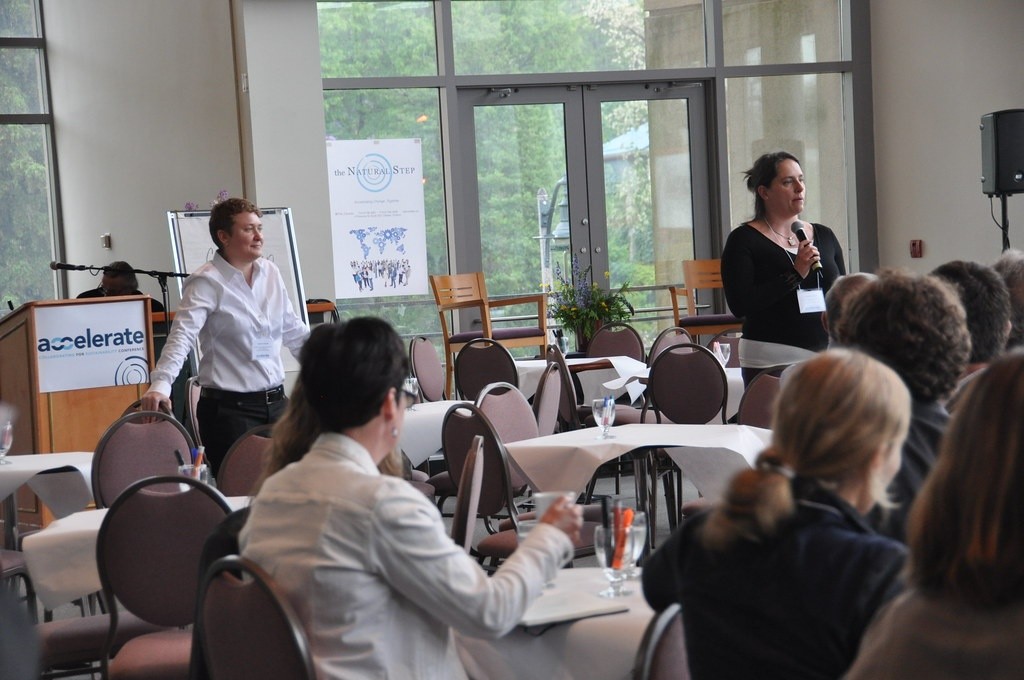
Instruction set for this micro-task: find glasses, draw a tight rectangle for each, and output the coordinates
[397,386,418,407]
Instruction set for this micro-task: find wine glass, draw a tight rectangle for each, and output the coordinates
[590,399,617,441]
[403,376,418,413]
[595,526,636,598]
[607,509,648,580]
[0,423,13,466]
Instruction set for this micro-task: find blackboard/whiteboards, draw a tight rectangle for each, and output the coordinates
[168,207,314,379]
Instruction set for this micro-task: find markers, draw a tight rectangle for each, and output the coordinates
[611,508,633,568]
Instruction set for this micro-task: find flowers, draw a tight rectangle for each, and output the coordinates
[538,252,636,343]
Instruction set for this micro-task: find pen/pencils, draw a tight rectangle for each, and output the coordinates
[192,445,205,480]
[613,498,621,542]
[173,450,188,476]
[601,496,612,565]
[553,329,565,352]
[602,396,613,427]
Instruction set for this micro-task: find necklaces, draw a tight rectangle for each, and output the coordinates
[771,226,798,246]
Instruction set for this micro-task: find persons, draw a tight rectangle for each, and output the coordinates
[840,268,970,538]
[139,198,314,473]
[821,270,882,344]
[235,320,585,680]
[99,262,171,363]
[274,315,412,487]
[936,253,1023,386]
[852,346,1024,680]
[643,346,913,680]
[718,150,847,388]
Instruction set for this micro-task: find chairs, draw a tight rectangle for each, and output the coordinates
[0,259,783,680]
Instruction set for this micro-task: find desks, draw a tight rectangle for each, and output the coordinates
[502,424,775,566]
[453,568,655,679]
[22,492,256,680]
[397,399,474,484]
[603,366,745,424]
[0,451,94,551]
[516,355,651,409]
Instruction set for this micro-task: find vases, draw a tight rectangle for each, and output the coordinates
[574,319,612,352]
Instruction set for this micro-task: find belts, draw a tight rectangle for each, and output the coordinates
[199,386,284,405]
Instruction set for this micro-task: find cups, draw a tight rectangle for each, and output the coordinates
[531,491,580,522]
[554,337,569,357]
[178,464,211,492]
[517,520,541,545]
[713,343,731,367]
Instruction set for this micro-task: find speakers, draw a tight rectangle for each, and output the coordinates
[980,108,1024,198]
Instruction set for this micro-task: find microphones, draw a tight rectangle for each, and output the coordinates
[791,221,823,278]
[50,261,86,270]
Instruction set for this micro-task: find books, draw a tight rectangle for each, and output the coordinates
[518,584,631,628]
